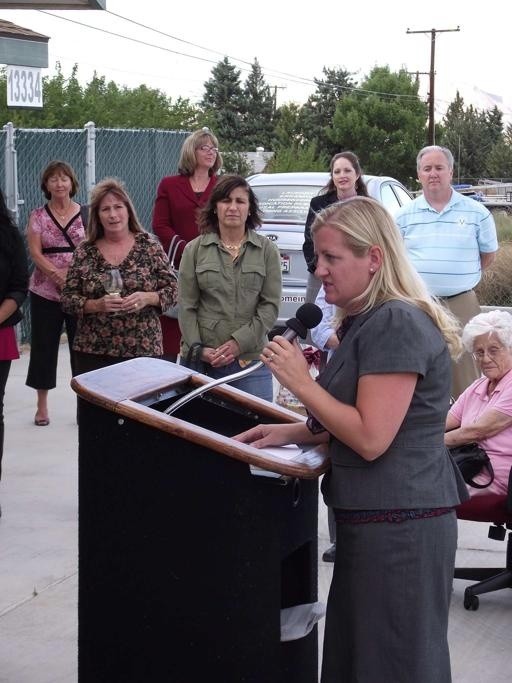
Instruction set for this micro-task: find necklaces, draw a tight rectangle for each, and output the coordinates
[191,177,209,190]
[50,198,72,220]
[221,238,243,251]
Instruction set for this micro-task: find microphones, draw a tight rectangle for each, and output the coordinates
[164,300,324,415]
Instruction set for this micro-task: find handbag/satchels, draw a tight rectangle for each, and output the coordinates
[161,234,187,319]
[449,442,495,489]
[276,342,326,416]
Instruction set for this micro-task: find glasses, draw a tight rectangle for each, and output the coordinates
[201,145,219,153]
[471,346,504,361]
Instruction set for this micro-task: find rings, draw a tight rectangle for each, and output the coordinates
[221,355,226,359]
[270,353,274,358]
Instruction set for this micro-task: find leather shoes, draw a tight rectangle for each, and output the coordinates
[322,544,337,562]
[35,410,49,425]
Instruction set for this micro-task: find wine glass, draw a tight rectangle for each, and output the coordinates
[103,269,127,318]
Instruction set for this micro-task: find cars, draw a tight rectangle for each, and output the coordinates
[452,183,485,202]
[243,171,414,330]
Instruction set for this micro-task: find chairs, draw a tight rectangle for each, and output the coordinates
[454,465,512,611]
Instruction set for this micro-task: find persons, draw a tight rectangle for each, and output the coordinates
[61,178,179,372]
[443,309,512,523]
[151,129,223,363]
[230,195,469,683]
[135,303,138,308]
[0,188,30,484]
[23,160,88,426]
[297,151,380,374]
[389,145,499,402]
[176,174,284,403]
[310,282,345,563]
